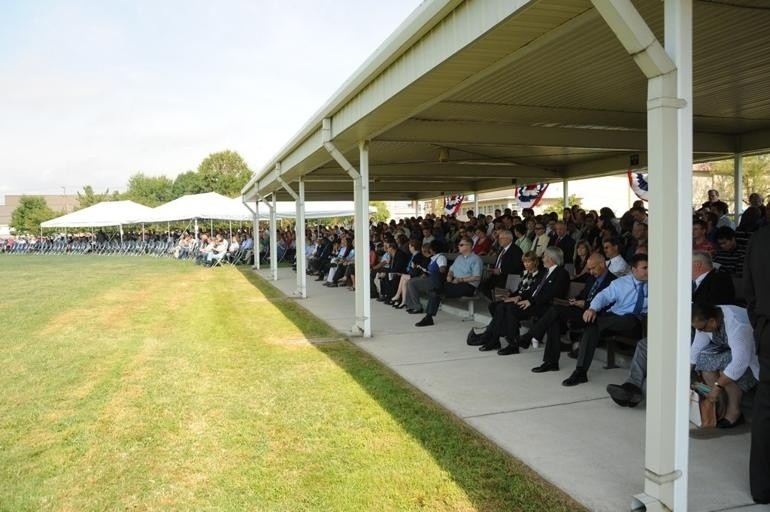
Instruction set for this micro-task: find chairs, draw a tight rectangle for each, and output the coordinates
[4,232,301,269]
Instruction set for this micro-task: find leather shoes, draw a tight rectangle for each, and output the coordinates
[416,316,433,325]
[717,413,745,429]
[506,335,530,349]
[533,363,558,372]
[563,371,587,385]
[607,382,642,407]
[480,338,500,351]
[498,344,519,354]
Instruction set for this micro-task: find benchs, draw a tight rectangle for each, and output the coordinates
[301,235,747,390]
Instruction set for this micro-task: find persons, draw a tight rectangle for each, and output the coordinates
[690,190,767,430]
[0,224,293,270]
[416,200,647,408]
[742,202,770,505]
[304,226,355,289]
[369,210,477,315]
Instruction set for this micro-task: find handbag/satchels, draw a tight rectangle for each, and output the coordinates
[690,382,717,429]
[468,326,492,345]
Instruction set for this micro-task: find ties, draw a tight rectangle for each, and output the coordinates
[633,284,644,314]
[497,249,505,268]
[534,238,539,252]
